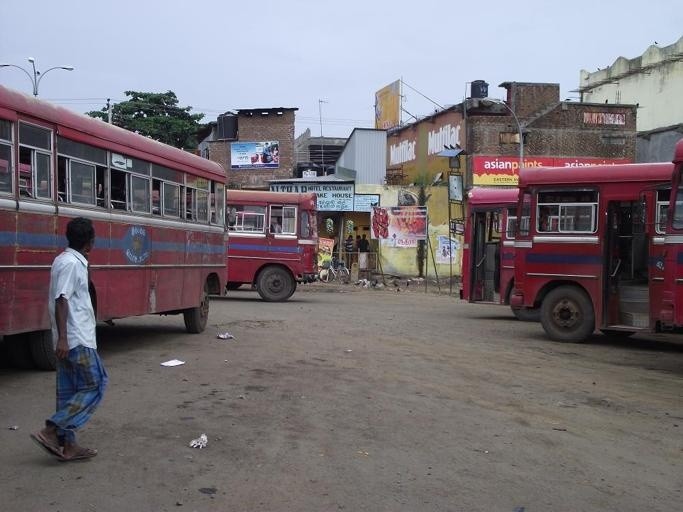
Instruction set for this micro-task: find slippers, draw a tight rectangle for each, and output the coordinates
[56,447,97,462]
[29,432,65,459]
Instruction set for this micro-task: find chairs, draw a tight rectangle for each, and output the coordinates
[233,210,257,231]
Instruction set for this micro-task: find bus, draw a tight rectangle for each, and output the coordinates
[225,188,322,303]
[0,83,229,373]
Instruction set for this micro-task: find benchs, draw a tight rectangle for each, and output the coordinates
[0,159,32,198]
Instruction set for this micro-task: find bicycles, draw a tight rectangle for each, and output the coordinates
[318,256,350,285]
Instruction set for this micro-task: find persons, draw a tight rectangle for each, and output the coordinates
[27,215,109,464]
[342,234,354,268]
[356,234,369,268]
[251,143,279,163]
[354,235,361,250]
[271,216,281,234]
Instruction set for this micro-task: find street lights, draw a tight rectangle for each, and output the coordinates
[481,95,525,167]
